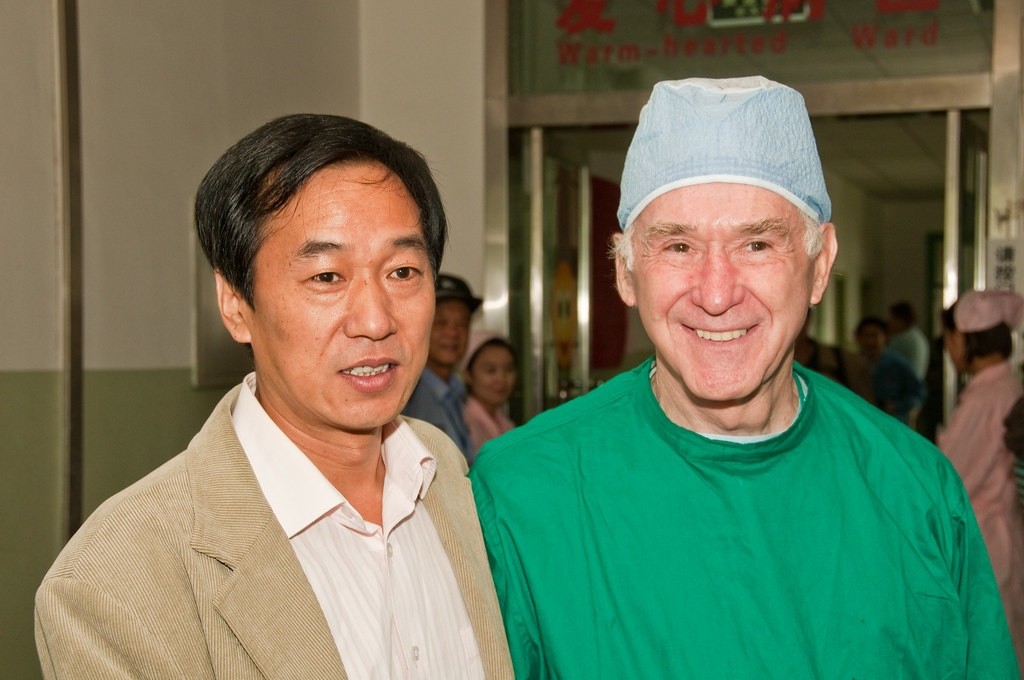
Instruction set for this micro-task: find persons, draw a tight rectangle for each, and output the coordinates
[402,272,1023,679]
[32,113,516,680]
[465,75,1023,680]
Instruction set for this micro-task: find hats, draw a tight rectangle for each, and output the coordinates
[953,290,1024,333]
[616,75,832,236]
[435,275,482,313]
[457,330,510,371]
